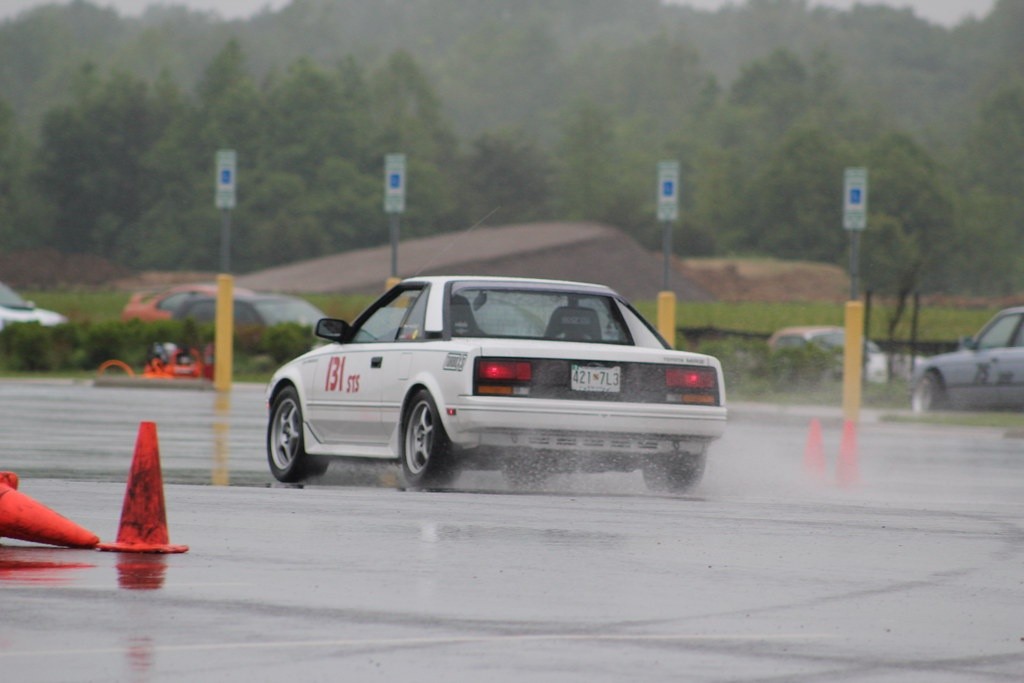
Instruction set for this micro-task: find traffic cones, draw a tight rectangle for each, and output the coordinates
[830,420,863,486]
[95,421,190,555]
[798,417,830,474]
[0,469,100,549]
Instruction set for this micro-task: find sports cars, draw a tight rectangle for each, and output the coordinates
[267,273,729,498]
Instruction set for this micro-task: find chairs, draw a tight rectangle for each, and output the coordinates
[450,296,486,337]
[543,306,601,341]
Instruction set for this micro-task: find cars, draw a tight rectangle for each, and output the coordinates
[909,303,1024,415]
[766,325,890,391]
[120,282,258,329]
[171,292,335,333]
[0,280,70,334]
